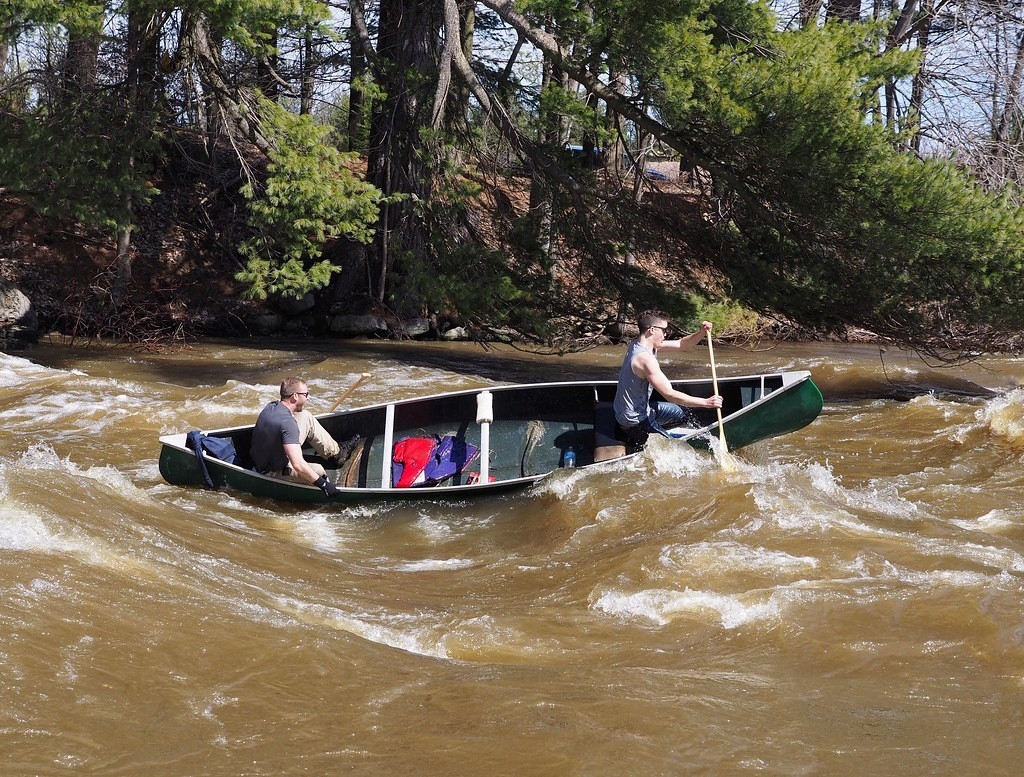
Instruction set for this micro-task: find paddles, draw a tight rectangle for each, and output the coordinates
[321,370,377,415]
[701,323,734,458]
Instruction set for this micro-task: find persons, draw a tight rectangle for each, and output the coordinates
[612,310,724,429]
[250,377,360,499]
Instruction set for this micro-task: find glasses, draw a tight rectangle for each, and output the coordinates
[289,392,308,398]
[647,326,668,332]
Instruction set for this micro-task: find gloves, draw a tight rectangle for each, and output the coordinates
[313,477,340,498]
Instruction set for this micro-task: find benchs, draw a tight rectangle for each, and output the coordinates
[593,389,626,464]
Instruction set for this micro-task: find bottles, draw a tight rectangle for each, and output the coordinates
[564,447,576,468]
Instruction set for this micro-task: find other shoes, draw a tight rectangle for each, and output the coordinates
[334,434,360,467]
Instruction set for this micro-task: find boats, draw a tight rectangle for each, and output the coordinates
[158,370,823,508]
